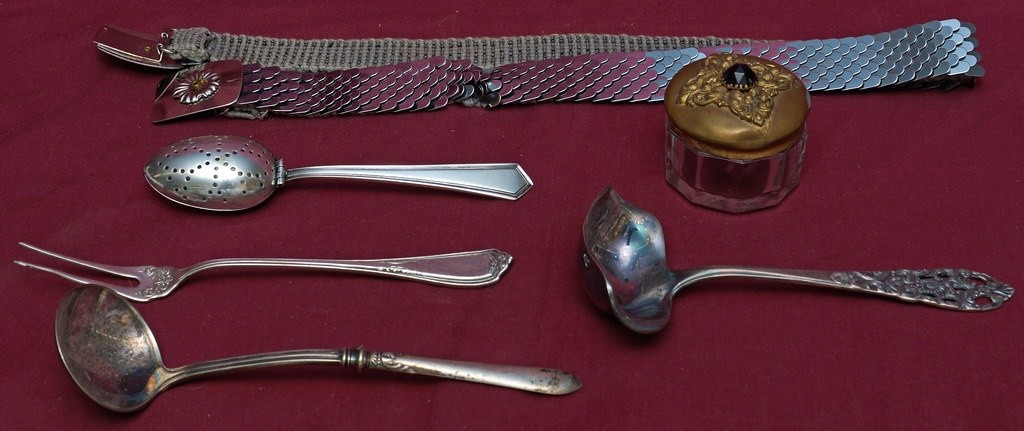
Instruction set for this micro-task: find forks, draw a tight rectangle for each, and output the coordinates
[14,238,512,303]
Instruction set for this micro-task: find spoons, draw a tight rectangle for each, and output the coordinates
[54,283,584,414]
[139,134,534,212]
[580,182,1014,336]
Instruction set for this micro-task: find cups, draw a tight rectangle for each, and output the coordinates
[662,50,812,212]
